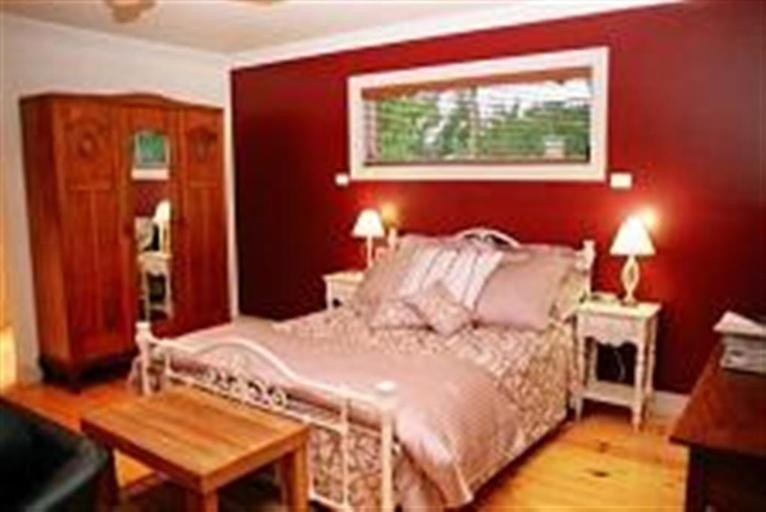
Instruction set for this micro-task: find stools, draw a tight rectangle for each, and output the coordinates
[82,385,309,512]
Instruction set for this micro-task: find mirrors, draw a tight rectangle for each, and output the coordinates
[129,127,175,327]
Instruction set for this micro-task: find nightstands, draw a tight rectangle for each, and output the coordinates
[324,271,365,310]
[576,297,664,431]
[138,250,175,323]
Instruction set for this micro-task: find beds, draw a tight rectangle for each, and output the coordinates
[132,226,597,512]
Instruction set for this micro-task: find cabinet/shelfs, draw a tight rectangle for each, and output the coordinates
[19,91,232,395]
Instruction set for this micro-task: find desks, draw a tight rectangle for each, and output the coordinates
[670,339,765,512]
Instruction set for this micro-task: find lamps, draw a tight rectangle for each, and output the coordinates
[608,218,657,305]
[150,198,171,250]
[350,210,385,273]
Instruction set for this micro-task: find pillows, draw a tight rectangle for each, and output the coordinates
[352,233,588,335]
